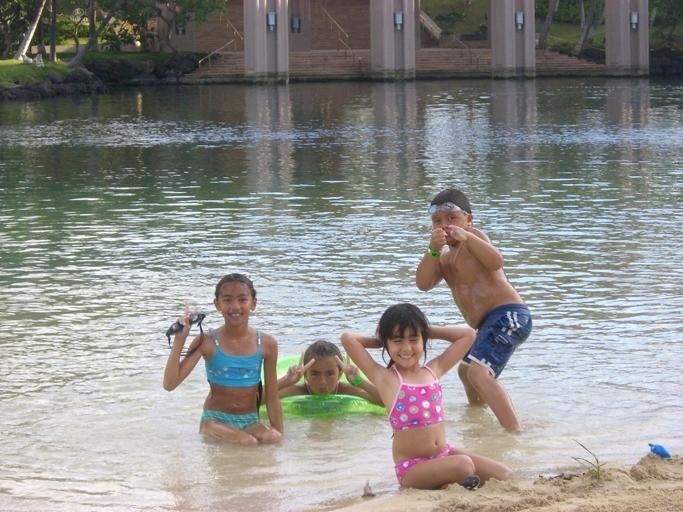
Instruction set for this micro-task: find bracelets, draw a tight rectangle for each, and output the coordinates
[349,374,364,388]
[427,246,443,258]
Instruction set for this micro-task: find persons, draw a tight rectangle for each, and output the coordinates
[260,339,386,409]
[342,303,516,488]
[163,274,284,446]
[415,188,533,434]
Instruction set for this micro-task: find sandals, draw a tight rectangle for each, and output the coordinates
[460,474,480,491]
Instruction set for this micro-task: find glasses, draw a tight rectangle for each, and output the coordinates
[166,312,205,357]
[427,203,469,216]
